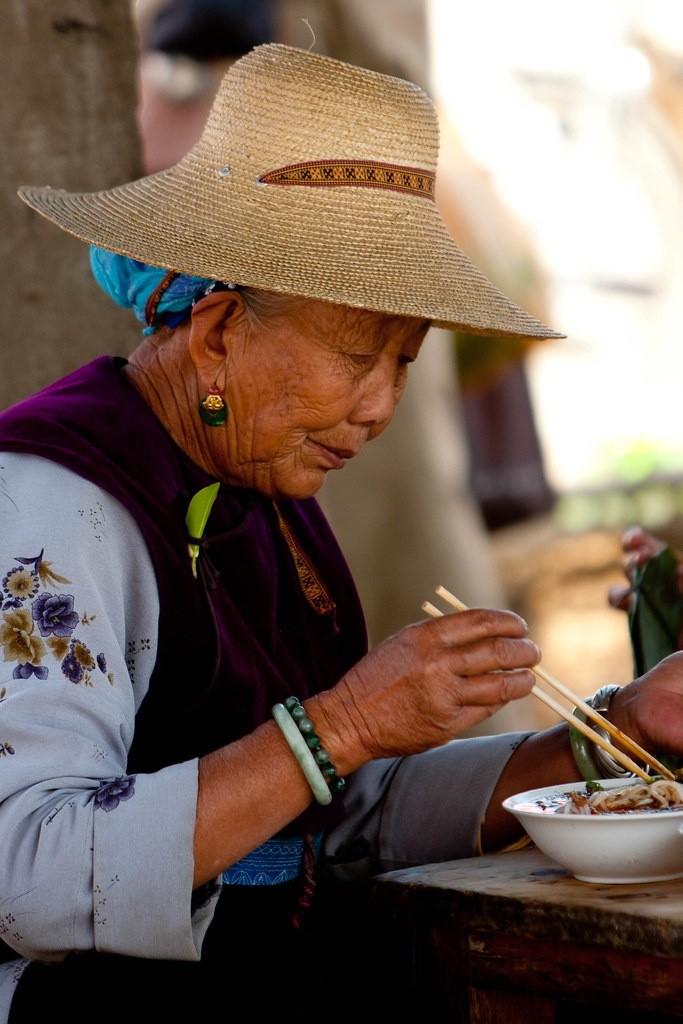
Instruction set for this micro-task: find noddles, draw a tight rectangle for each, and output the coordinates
[533,770,683,816]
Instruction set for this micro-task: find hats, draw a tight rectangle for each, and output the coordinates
[16,43,568,342]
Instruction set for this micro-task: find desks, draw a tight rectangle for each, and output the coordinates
[375,842,683,1023]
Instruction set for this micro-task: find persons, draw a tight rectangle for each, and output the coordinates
[606,525,683,611]
[128,0,278,175]
[0,43,682,1024]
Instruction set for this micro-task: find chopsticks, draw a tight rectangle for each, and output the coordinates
[421,586,675,783]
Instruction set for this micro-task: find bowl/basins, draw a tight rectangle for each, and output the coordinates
[501,776,683,883]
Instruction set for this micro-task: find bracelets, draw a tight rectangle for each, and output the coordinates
[570,681,653,780]
[273,695,347,804]
